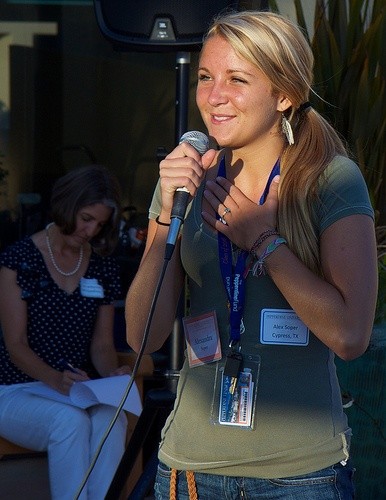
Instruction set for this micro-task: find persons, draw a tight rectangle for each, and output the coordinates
[1,165,132,499]
[125,10,380,500]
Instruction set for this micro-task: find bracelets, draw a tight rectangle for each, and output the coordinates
[252,237,288,279]
[249,229,281,258]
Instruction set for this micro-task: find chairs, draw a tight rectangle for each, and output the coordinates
[0,349,154,500]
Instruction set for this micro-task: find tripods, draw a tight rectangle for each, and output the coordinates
[101,57,193,500]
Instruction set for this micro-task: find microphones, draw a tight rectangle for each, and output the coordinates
[164,131,210,259]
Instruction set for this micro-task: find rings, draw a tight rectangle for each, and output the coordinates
[219,208,231,225]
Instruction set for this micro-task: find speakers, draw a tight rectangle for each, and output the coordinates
[93,0,238,53]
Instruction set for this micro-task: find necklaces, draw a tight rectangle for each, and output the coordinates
[44,222,84,276]
